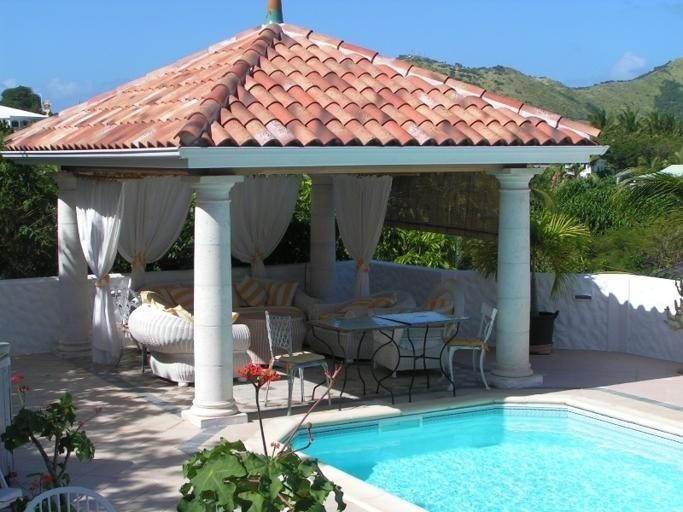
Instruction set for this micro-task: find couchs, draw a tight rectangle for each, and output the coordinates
[127,277,326,390]
[373,279,468,379]
[303,288,418,364]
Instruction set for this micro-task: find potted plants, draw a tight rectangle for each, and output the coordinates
[473,188,593,354]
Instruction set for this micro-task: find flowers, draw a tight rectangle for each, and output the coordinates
[234,361,281,389]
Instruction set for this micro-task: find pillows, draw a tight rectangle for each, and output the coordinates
[140,286,177,311]
[427,294,448,312]
[319,292,399,321]
[236,276,264,306]
[266,279,300,305]
[168,284,194,314]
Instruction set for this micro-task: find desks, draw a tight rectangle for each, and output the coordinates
[310,309,471,409]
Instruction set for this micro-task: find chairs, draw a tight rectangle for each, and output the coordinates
[24,486,119,512]
[109,287,147,375]
[440,301,500,389]
[263,308,332,417]
